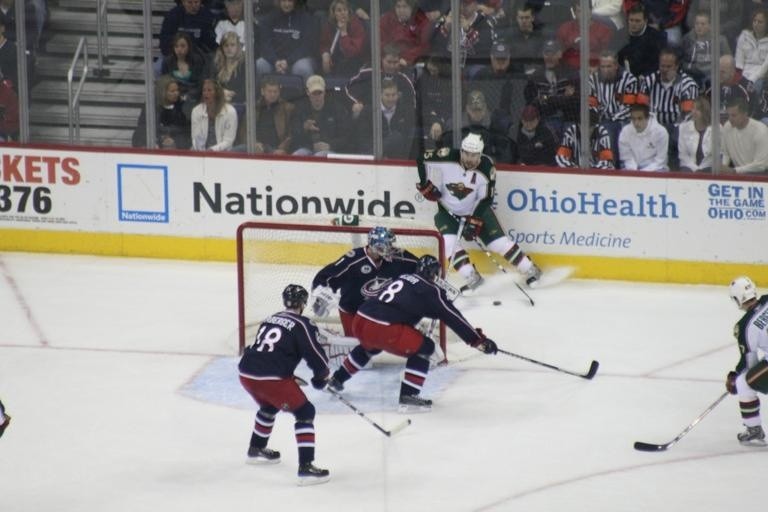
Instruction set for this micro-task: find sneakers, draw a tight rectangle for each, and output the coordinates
[299,462,328,477]
[328,375,344,392]
[738,425,764,441]
[399,395,432,406]
[248,446,280,460]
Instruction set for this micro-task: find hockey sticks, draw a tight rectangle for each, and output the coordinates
[635,391,730,452]
[328,389,411,436]
[425,216,466,360]
[436,198,534,308]
[497,348,598,379]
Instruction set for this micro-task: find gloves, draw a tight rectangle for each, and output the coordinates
[314,297,328,316]
[463,215,483,241]
[312,378,327,390]
[471,328,497,355]
[727,372,738,394]
[416,180,441,201]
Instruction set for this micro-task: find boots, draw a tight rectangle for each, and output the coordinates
[527,256,541,284]
[460,264,481,292]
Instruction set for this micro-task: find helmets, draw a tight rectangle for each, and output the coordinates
[283,284,308,307]
[414,255,440,281]
[460,132,484,162]
[730,276,757,309]
[368,226,397,256]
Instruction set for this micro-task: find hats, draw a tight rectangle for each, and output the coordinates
[491,43,510,58]
[467,91,485,110]
[524,104,539,120]
[306,75,326,93]
[544,40,559,52]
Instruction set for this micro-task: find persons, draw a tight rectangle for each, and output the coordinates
[238,283,331,476]
[416,132,541,290]
[131,2,768,173]
[0,403,10,437]
[726,276,768,441]
[326,255,496,406]
[2,0,36,145]
[310,226,422,355]
